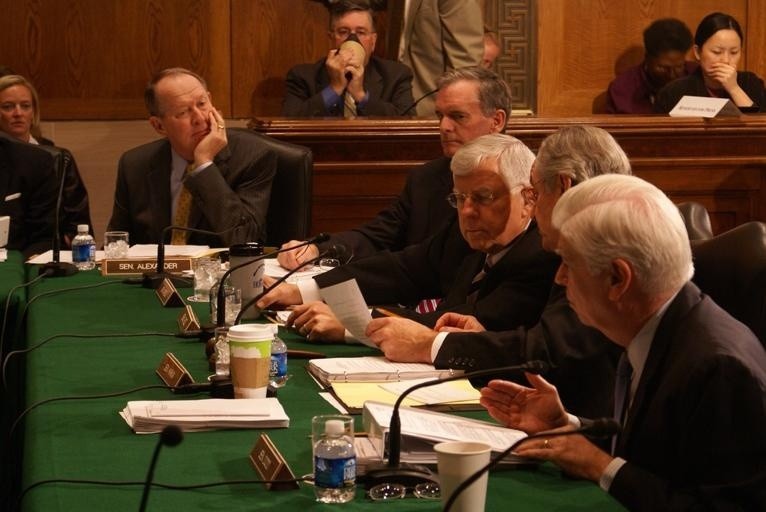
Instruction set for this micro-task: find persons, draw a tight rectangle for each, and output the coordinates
[104,66,277,262]
[406,0,486,119]
[601,17,712,115]
[276,64,512,317]
[0,75,89,251]
[281,3,418,118]
[254,131,563,355]
[366,126,633,409]
[653,12,766,113]
[480,23,502,72]
[477,171,765,512]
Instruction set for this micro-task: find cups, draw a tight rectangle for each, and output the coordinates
[104,231,128,259]
[312,414,354,474]
[338,34,367,75]
[214,326,230,377]
[228,323,273,399]
[216,286,242,326]
[433,441,491,511]
[194,258,221,301]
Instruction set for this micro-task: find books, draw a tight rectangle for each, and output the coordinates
[120,396,289,434]
[126,242,210,260]
[308,351,492,415]
[360,397,538,467]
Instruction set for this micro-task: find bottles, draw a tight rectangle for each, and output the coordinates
[269,324,287,387]
[71,224,97,271]
[315,421,357,502]
[229,242,264,319]
[211,271,234,323]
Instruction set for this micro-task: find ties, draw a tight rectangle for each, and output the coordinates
[611,353,632,456]
[466,254,492,307]
[170,163,197,246]
[343,86,358,119]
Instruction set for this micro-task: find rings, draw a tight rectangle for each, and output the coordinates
[217,125,225,130]
[302,323,308,334]
[544,439,549,449]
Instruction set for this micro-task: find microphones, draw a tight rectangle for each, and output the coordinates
[443,418,622,512]
[39,151,79,278]
[139,425,183,511]
[142,216,250,290]
[366,359,550,487]
[200,231,331,340]
[209,244,346,398]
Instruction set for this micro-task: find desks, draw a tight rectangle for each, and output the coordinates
[3,248,32,303]
[31,246,638,509]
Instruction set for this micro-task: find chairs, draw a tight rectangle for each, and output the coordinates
[667,200,766,347]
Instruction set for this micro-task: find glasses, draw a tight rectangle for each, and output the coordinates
[330,29,376,41]
[447,186,493,208]
[522,177,552,203]
[365,484,442,501]
